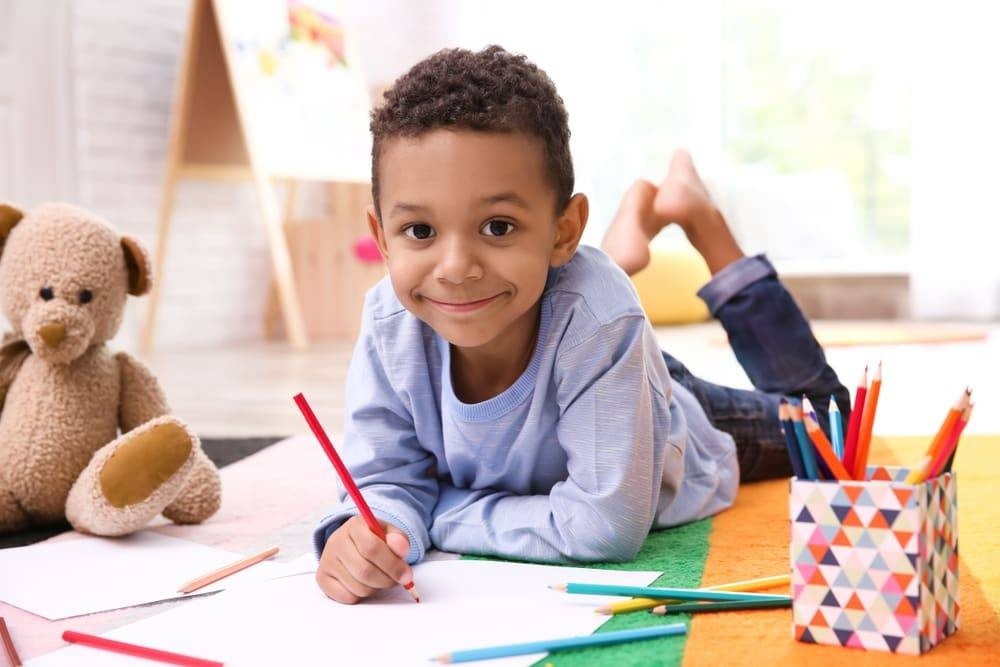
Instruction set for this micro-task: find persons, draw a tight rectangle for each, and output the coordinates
[312,47,851,605]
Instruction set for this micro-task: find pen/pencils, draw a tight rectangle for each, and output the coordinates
[292,391,424,604]
[428,622,688,664]
[547,583,792,602]
[778,360,883,483]
[647,598,790,616]
[909,387,977,486]
[595,574,791,615]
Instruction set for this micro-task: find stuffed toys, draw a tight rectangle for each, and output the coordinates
[0,204,220,539]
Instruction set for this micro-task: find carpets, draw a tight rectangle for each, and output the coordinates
[0,433,1000,667]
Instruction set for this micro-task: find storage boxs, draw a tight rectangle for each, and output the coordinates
[788,464,961,657]
